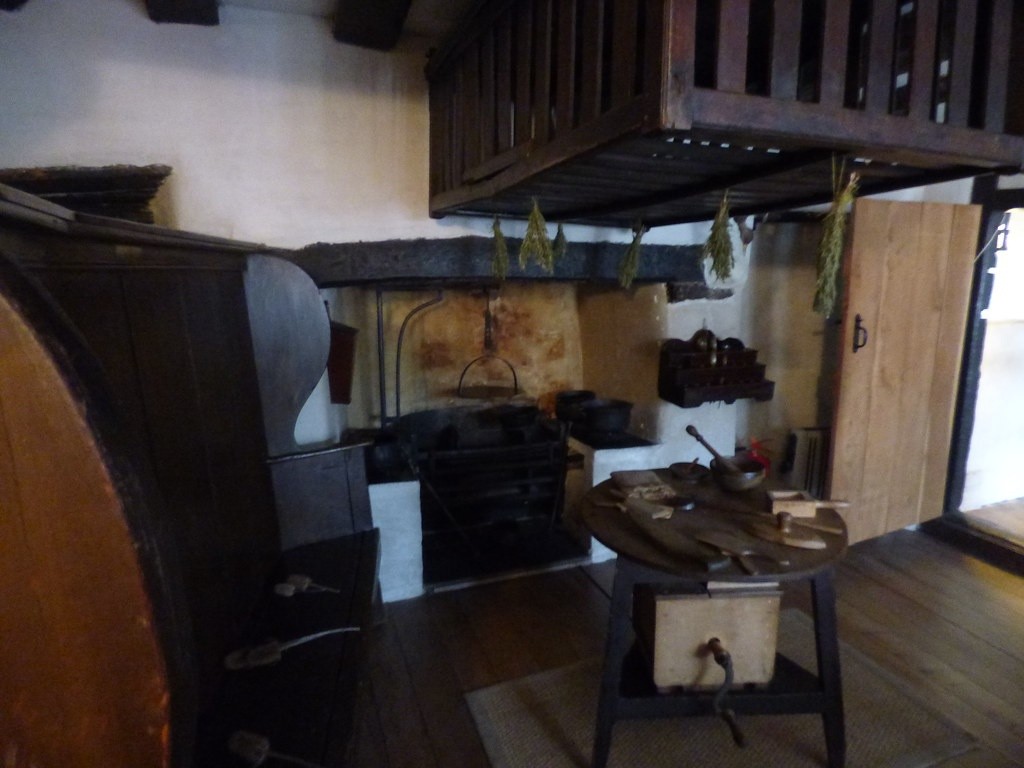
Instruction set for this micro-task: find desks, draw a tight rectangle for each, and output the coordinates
[577,466,849,768]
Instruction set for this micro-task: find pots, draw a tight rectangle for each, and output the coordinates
[387,407,459,449]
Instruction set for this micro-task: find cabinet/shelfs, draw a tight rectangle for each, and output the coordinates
[656,329,776,409]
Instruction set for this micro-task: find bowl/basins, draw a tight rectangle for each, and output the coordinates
[670,461,708,484]
[714,457,767,492]
[582,400,635,434]
[553,389,595,423]
